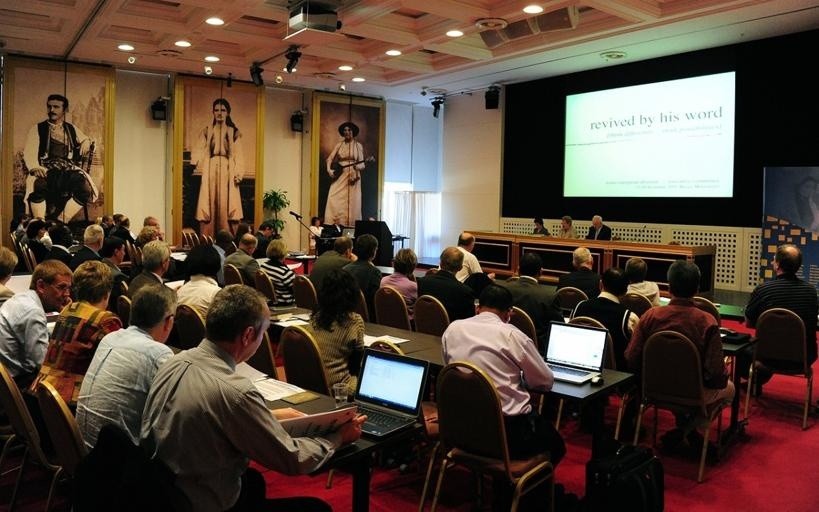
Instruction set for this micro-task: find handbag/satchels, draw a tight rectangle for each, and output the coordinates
[583,443,665,512]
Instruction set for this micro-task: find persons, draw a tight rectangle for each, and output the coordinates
[324,122,376,228]
[21,94,99,226]
[623,255,661,309]
[621,260,728,468]
[190,98,245,224]
[740,243,819,393]
[570,269,647,444]
[553,246,600,299]
[558,216,579,240]
[778,167,819,232]
[584,215,611,240]
[440,285,567,511]
[532,216,552,237]
[501,251,573,429]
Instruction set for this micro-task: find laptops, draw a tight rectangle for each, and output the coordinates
[539,319,610,387]
[327,347,431,440]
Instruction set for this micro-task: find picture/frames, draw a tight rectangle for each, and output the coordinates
[299,89,386,255]
[0,52,117,274]
[173,71,267,247]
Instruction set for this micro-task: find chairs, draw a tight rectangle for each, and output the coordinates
[359,266,758,511]
[743,306,819,431]
[0,221,370,511]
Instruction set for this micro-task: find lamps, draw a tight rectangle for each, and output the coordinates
[276,76,283,83]
[290,110,304,132]
[204,65,213,74]
[339,84,346,91]
[249,61,265,87]
[285,44,302,74]
[151,96,171,120]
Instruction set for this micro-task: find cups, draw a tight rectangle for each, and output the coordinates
[333,383,348,407]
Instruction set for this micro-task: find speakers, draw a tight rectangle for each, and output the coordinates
[291,115,303,132]
[484,90,499,109]
[151,101,168,120]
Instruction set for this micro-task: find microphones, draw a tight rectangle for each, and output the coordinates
[289,210,303,219]
[637,225,647,242]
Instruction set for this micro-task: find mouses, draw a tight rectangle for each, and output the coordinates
[591,376,604,386]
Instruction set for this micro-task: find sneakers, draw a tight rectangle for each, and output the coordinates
[739,382,762,398]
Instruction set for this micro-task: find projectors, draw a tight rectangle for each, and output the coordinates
[289,4,343,32]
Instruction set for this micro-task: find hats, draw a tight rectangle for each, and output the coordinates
[338,122,360,138]
